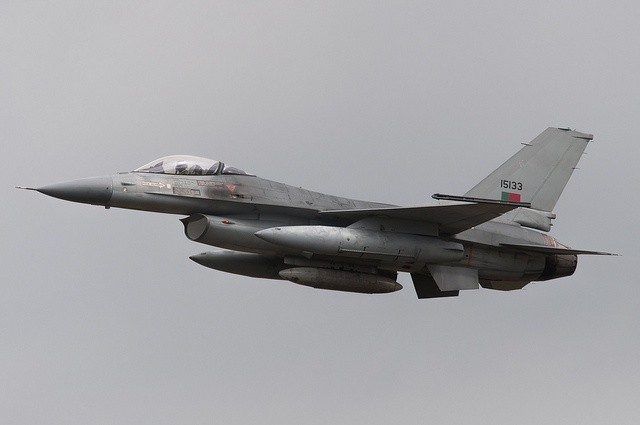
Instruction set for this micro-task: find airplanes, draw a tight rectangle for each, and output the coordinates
[15,125,622,299]
